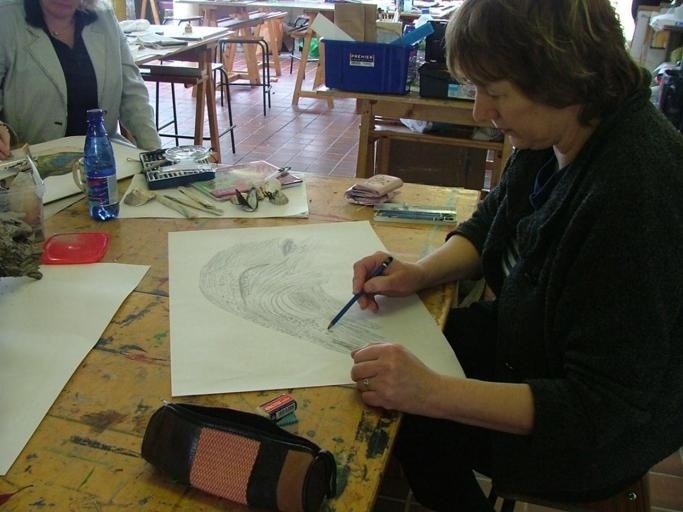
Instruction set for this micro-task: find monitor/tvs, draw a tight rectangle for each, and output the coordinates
[189,158,303,202]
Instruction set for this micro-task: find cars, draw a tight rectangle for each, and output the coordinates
[71,158,87,196]
[0,179,47,251]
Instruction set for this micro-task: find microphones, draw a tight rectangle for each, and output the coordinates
[112,1,312,165]
[484,477,649,510]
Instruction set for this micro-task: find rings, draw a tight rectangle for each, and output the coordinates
[328,257,393,329]
[377,211,453,221]
[377,6,400,22]
[4,151,15,157]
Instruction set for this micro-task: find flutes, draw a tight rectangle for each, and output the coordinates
[373,203,457,227]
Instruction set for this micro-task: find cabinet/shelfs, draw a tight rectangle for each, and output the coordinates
[83,109,120,223]
[416,9,432,86]
[184,24,191,33]
[402,25,418,83]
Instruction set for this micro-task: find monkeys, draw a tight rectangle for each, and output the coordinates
[256,393,297,422]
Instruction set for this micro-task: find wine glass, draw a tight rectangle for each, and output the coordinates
[362,378,369,391]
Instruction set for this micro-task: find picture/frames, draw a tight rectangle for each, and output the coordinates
[0,161,480,512]
[316,79,513,200]
[289,5,445,108]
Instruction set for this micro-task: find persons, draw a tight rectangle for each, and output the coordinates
[0,0,161,160]
[351,1,683,509]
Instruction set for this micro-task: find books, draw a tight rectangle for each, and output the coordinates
[416,16,481,102]
[319,38,419,96]
